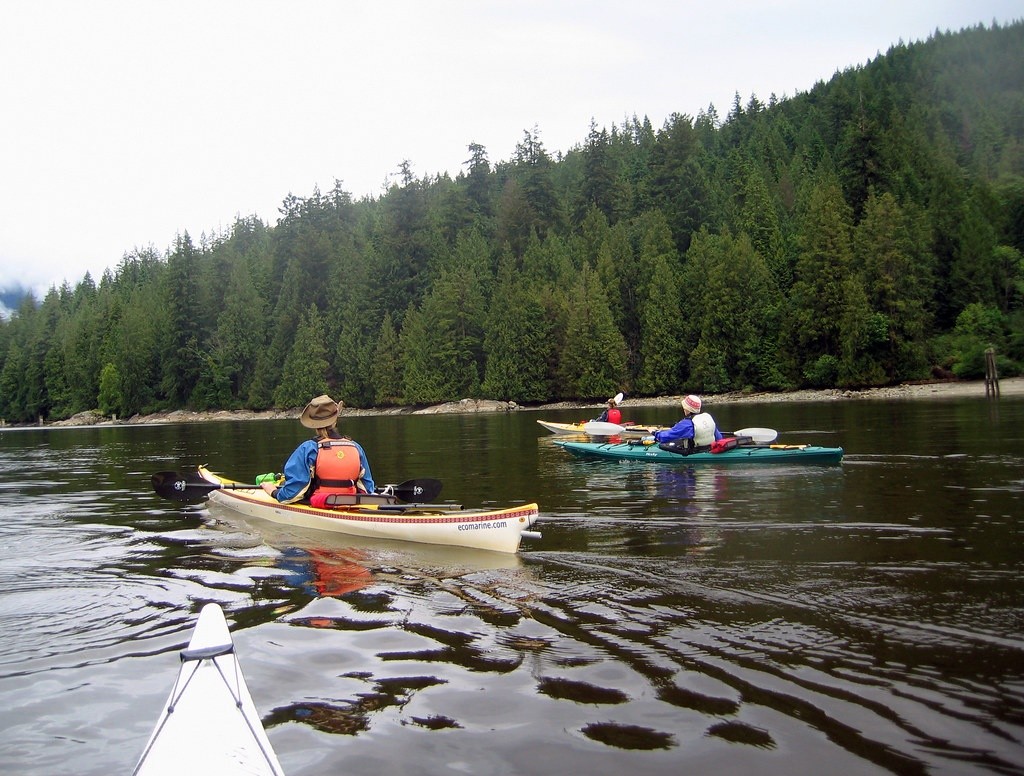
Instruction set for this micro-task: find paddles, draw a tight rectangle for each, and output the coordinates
[150,469,443,504]
[339,504,464,510]
[738,444,807,449]
[582,392,623,434]
[584,421,778,442]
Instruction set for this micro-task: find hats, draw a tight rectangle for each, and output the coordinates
[681,395,701,413]
[605,398,615,404]
[300,395,344,429]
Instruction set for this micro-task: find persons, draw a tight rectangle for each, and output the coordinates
[260,395,375,505]
[647,395,722,447]
[596,398,621,425]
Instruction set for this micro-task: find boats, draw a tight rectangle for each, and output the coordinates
[197,464,541,553]
[551,437,844,467]
[537,420,672,438]
